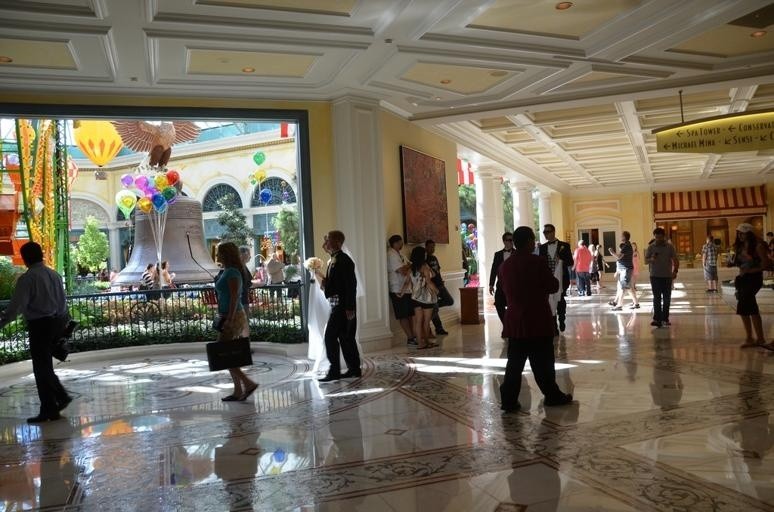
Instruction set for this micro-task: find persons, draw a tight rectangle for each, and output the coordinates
[306,230,362,381]
[213,243,260,401]
[0,242,74,423]
[267,253,285,300]
[489,224,610,411]
[387,234,453,350]
[142,261,176,299]
[608,223,774,349]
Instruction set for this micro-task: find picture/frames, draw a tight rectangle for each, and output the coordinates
[399,145,450,245]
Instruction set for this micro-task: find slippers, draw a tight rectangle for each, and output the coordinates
[222,383,257,402]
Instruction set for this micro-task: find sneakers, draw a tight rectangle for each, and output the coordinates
[608,301,623,310]
[407,328,448,349]
[706,288,718,293]
[652,318,672,327]
[28,393,71,422]
[631,304,641,309]
[501,399,522,410]
[558,320,565,331]
[545,392,573,406]
[740,338,774,351]
[319,368,362,381]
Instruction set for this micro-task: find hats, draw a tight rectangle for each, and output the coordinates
[736,223,752,232]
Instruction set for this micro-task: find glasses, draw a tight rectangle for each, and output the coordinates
[543,230,551,235]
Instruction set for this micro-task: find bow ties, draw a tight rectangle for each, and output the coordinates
[548,242,555,245]
[503,249,511,252]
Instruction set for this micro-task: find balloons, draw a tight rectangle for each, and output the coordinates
[461,223,478,254]
[115,170,183,221]
[248,152,288,204]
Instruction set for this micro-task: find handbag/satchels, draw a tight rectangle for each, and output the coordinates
[206,336,253,371]
